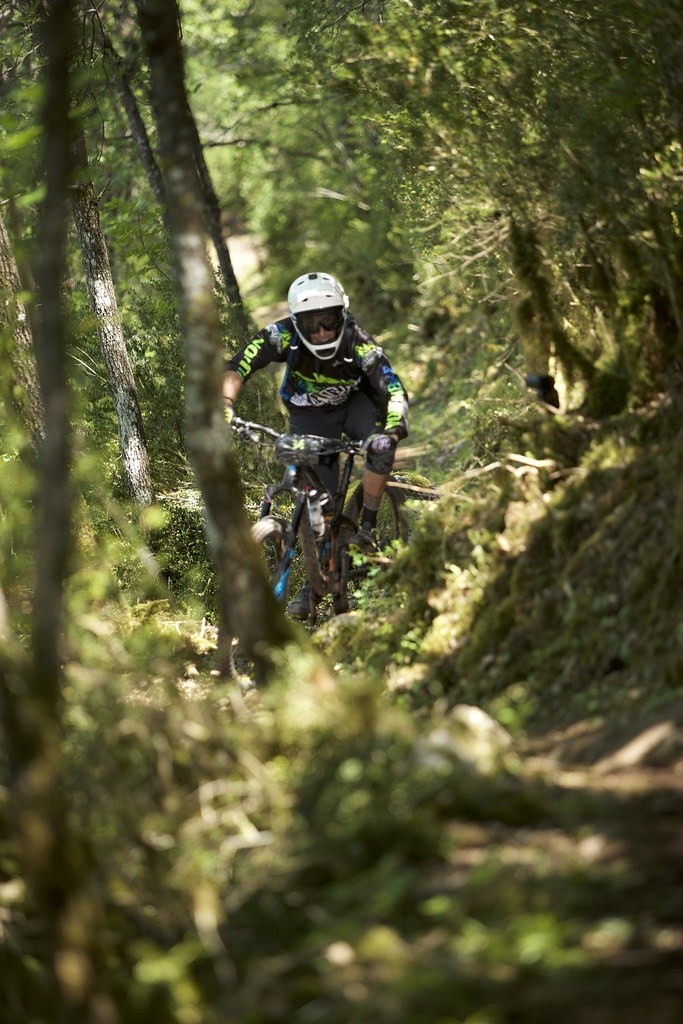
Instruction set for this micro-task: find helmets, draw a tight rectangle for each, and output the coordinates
[286,272,349,360]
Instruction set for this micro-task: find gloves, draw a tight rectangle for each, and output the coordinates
[359,434,396,456]
[223,406,236,426]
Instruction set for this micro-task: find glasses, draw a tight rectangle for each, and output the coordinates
[296,309,340,334]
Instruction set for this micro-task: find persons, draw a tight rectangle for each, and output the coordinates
[220,268,411,619]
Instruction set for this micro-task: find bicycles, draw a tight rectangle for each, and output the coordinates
[213,414,402,690]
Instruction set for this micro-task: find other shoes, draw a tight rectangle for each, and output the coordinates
[348,530,377,554]
[287,586,322,619]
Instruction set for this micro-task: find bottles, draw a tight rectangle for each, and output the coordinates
[306,486,325,537]
